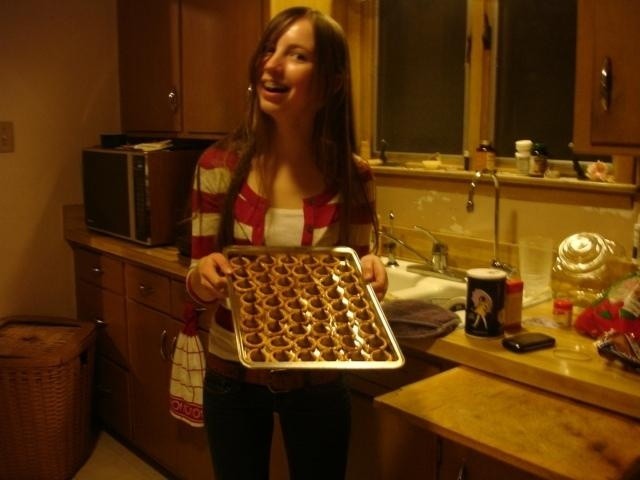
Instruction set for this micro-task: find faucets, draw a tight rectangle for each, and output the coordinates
[377,224,449,274]
[387,210,398,267]
[466,169,500,261]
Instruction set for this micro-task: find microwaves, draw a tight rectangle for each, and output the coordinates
[79,143,188,249]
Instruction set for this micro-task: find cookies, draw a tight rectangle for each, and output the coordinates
[229,253,393,362]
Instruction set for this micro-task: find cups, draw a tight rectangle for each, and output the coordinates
[465,268,507,341]
[516,235,555,296]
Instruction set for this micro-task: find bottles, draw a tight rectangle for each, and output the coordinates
[501,278,524,336]
[359,140,370,165]
[531,143,547,177]
[475,139,497,175]
[554,299,572,330]
[514,139,535,174]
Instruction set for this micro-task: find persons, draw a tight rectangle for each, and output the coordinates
[180,4,390,480]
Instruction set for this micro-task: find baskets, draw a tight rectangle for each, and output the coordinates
[0,325,97,480]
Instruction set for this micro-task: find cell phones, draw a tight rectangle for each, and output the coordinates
[502,332,555,352]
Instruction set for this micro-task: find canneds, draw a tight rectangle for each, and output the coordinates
[476,139,496,171]
[529,143,545,177]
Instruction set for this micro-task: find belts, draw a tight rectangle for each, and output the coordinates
[207,353,341,393]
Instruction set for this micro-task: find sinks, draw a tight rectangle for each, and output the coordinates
[385,268,419,294]
[415,286,467,328]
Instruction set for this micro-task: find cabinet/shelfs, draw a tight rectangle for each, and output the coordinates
[65,228,234,480]
[116,0,276,138]
[570,0,640,157]
